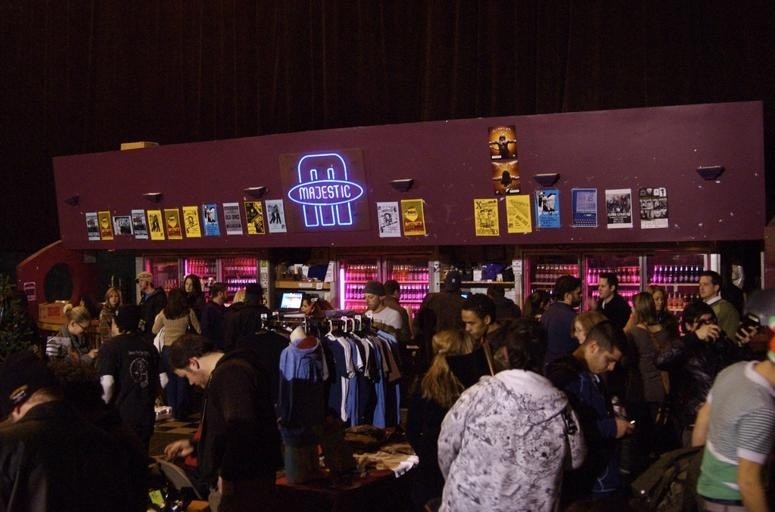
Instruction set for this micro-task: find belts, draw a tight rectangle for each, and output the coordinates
[702,495,742,505]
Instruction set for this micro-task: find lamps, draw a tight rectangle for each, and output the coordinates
[534,173,559,186]
[390,179,413,192]
[245,187,265,199]
[699,165,721,180]
[142,192,160,201]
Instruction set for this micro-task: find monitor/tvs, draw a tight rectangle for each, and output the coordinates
[279,292,305,311]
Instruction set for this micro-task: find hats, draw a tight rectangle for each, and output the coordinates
[552,276,582,296]
[445,270,461,291]
[0,349,65,421]
[365,281,385,295]
[134,272,154,284]
[111,303,141,330]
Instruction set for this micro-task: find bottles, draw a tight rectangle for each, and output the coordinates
[527,262,704,316]
[150,255,260,299]
[339,261,430,315]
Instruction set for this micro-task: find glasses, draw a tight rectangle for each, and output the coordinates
[77,323,89,330]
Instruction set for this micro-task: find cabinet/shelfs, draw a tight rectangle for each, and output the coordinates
[642,255,709,331]
[144,256,178,293]
[523,251,642,312]
[222,257,257,297]
[338,255,429,320]
[185,258,217,301]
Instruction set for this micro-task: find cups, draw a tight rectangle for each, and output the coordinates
[445,263,508,281]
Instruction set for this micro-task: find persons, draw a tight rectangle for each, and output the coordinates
[403,272,775,512]
[1,273,413,512]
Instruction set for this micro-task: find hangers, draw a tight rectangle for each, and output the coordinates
[261,315,398,359]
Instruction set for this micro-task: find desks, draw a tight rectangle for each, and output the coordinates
[276,443,417,511]
